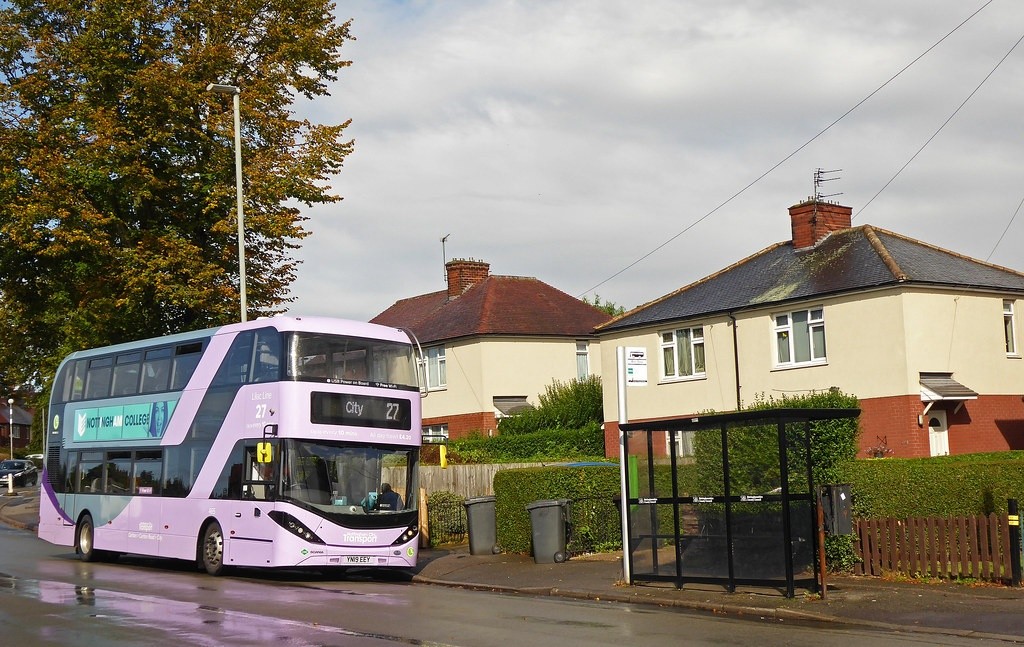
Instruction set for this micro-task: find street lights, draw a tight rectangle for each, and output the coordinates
[205,83,249,323]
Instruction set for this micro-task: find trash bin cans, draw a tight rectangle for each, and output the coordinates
[463,496,501,555]
[526,499,573,563]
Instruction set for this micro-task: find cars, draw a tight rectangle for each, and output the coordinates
[0,459,39,488]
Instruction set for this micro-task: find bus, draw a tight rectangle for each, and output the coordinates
[36,315,451,586]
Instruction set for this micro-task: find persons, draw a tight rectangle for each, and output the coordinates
[371,482,405,511]
[149,401,169,437]
